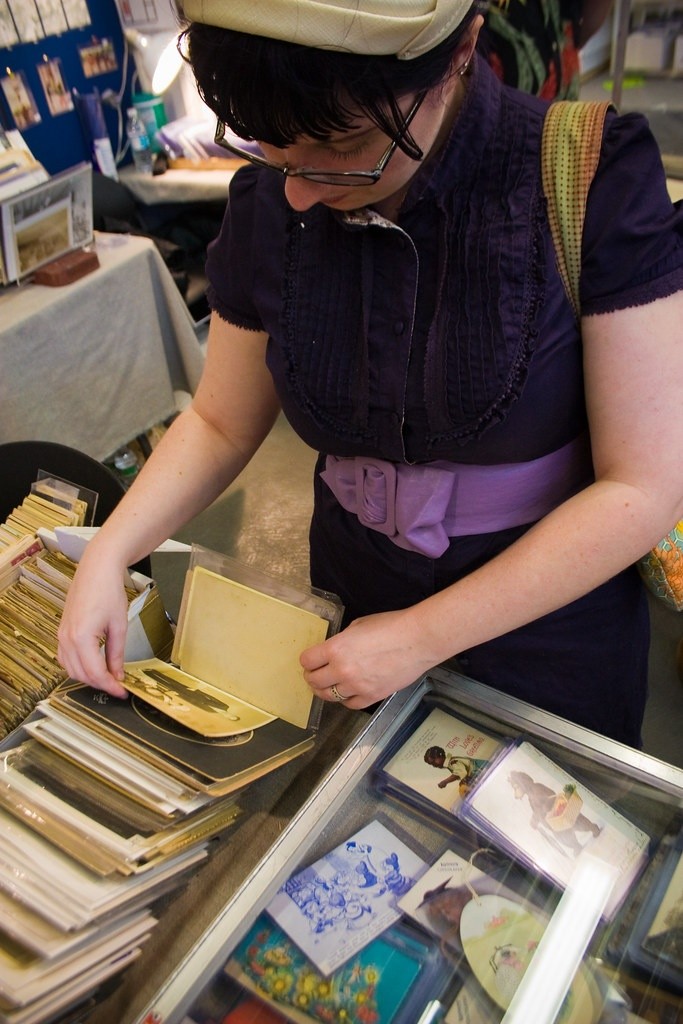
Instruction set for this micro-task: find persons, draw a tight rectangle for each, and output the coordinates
[125,668,240,722]
[423,746,491,789]
[57,0,683,804]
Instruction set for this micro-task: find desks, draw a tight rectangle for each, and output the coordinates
[118,163,236,234]
[1,229,206,464]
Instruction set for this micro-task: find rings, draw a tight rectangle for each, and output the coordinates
[330,685,348,701]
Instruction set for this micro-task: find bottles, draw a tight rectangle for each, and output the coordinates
[113,446,142,485]
[126,106,153,172]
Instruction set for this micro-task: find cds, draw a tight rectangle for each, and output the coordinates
[460,898,606,1024]
[129,693,255,746]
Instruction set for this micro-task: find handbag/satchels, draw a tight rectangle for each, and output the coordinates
[638,518,683,612]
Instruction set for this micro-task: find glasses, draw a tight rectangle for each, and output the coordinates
[214,85,431,187]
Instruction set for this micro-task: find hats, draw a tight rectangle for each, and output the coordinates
[181,1,473,60]
